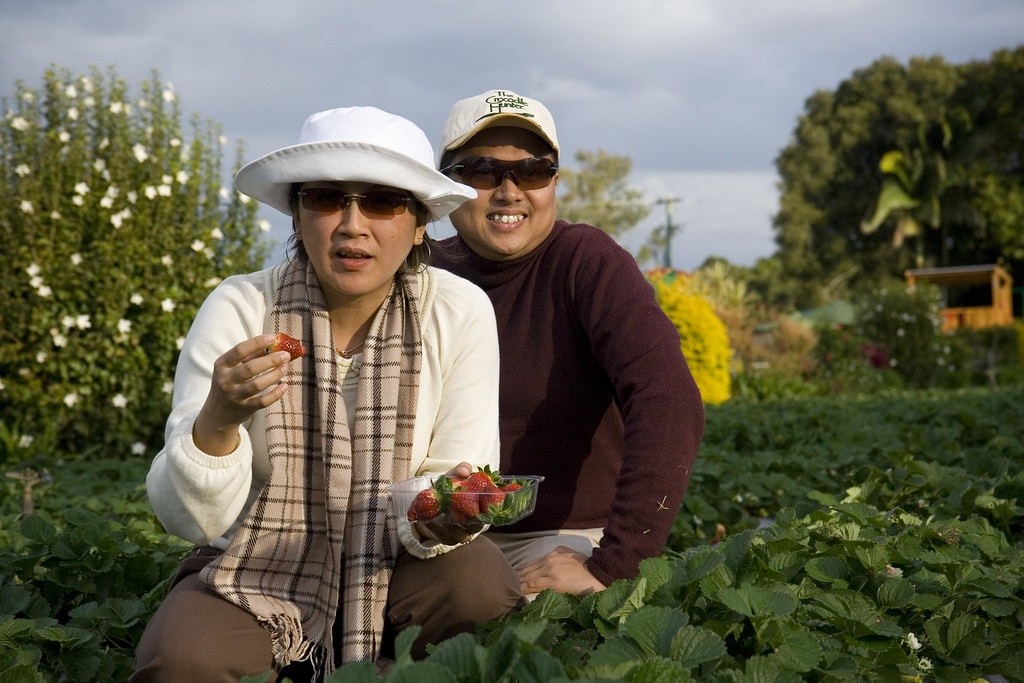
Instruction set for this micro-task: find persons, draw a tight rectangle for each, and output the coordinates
[127,105,530,683]
[428,89,704,602]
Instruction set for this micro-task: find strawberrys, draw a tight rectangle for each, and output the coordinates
[263,333,305,362]
[406,464,533,522]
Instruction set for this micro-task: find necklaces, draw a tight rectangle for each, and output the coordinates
[337,341,364,358]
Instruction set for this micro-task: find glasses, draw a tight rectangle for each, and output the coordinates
[440,156,560,186]
[299,188,412,215]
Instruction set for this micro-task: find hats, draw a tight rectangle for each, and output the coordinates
[442,89,560,162]
[235,107,478,223]
